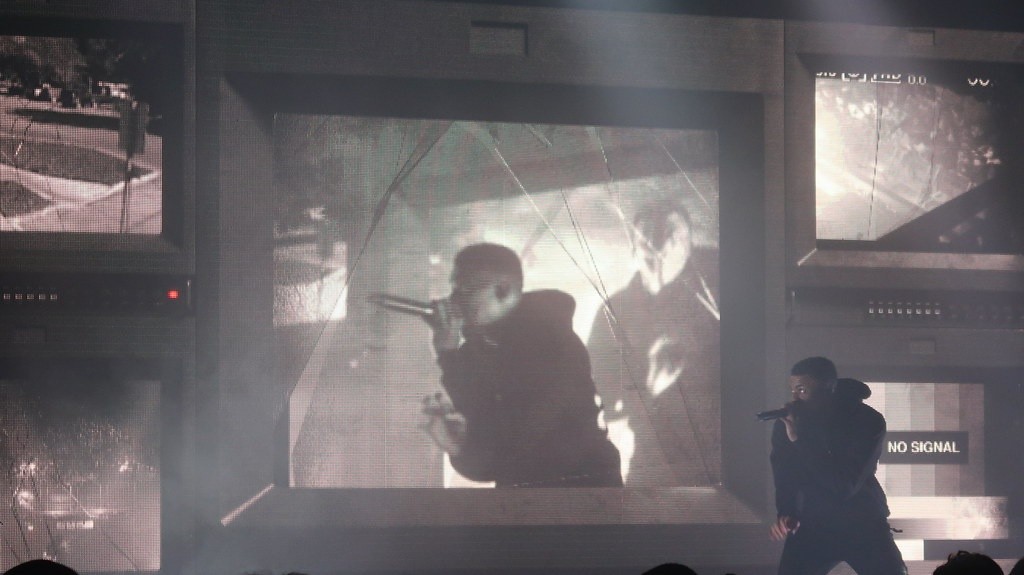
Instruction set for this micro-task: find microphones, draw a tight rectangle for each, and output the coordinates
[757,408,789,421]
[380,295,436,317]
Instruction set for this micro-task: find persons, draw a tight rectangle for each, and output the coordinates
[2,558,80,575]
[366,242,622,488]
[755,355,908,573]
[642,562,698,575]
[585,201,722,488]
[932,550,1024,574]
